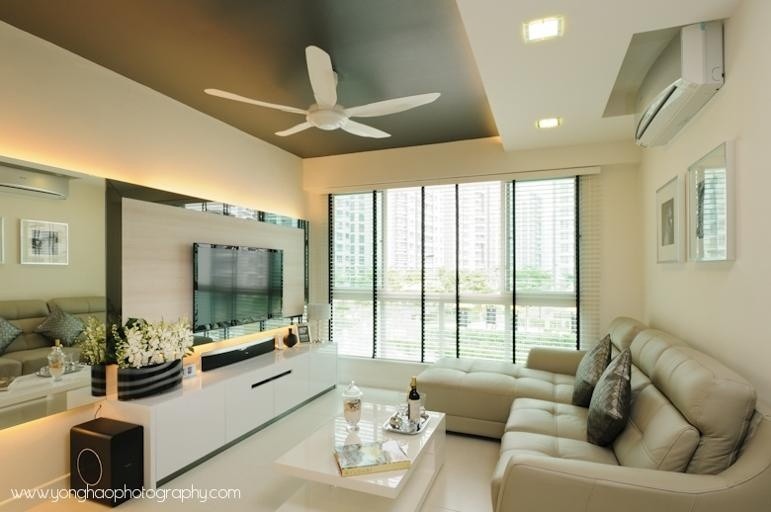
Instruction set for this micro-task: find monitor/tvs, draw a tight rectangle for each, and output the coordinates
[193,243,283,333]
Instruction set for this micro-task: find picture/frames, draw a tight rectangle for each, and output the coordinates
[650,172,684,267]
[683,137,736,263]
[18,217,69,266]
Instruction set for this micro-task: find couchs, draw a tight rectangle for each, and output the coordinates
[0,294,109,379]
[412,315,638,445]
[491,323,770,512]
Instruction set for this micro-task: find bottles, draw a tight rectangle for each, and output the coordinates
[283,327,298,347]
[408,377,421,425]
[39,339,78,379]
[340,380,363,433]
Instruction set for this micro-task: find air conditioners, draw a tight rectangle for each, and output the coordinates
[633,18,727,149]
[0,164,69,200]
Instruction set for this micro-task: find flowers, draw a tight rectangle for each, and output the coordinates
[78,316,105,366]
[108,314,197,365]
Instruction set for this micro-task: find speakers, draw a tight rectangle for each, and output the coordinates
[201,336,275,372]
[70,417,144,508]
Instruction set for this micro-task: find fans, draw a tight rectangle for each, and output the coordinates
[202,43,443,141]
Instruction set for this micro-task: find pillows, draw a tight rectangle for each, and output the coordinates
[0,317,20,357]
[33,304,82,349]
[573,331,611,406]
[584,347,632,444]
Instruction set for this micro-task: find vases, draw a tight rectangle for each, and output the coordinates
[282,326,298,349]
[114,359,184,402]
[89,363,105,398]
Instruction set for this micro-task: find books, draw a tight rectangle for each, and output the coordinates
[333,439,411,479]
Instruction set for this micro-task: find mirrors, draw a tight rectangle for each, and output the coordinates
[0,154,109,431]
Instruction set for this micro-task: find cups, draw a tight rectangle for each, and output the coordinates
[390,392,428,431]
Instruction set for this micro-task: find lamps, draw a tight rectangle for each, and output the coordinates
[308,303,330,344]
